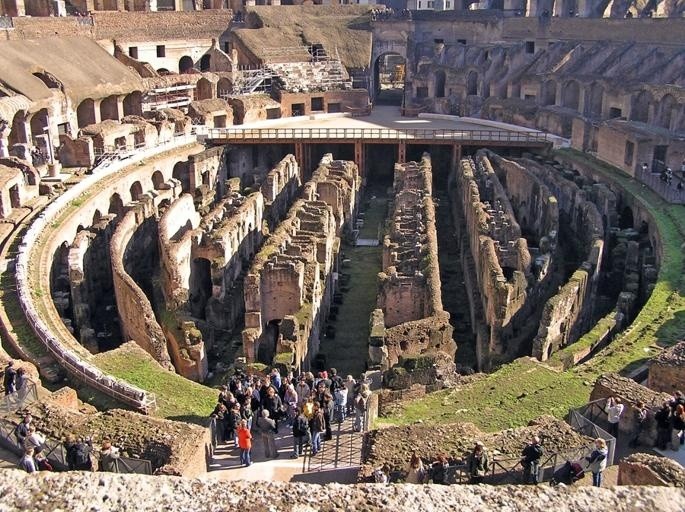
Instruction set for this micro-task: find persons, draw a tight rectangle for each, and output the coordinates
[588,438,609,487]
[641,161,685,192]
[15,414,121,472]
[521,436,544,485]
[367,437,489,486]
[605,390,685,451]
[4,361,27,402]
[210,366,370,467]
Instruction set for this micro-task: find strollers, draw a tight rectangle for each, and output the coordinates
[549,454,591,488]
